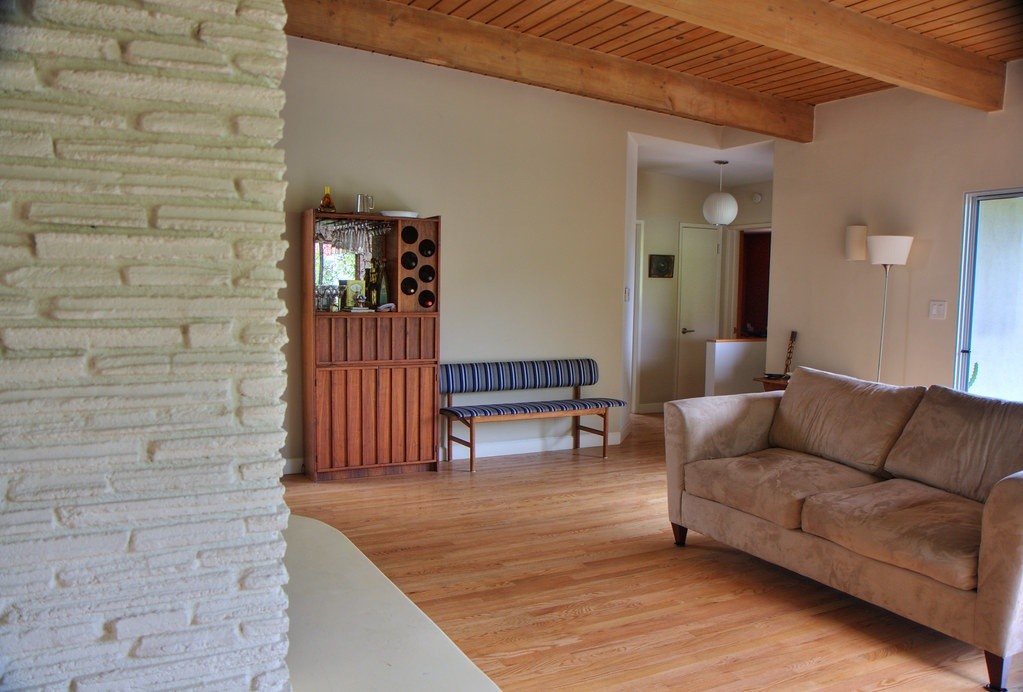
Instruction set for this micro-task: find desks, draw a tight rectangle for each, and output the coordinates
[752,377,789,391]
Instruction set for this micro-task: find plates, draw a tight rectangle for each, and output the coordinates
[379,210,420,218]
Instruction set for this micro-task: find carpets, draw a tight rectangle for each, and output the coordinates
[281,515,502,691]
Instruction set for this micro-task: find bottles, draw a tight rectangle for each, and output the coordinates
[318,185,337,213]
[368,259,391,308]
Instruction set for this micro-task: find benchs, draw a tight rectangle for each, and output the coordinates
[439,358,628,473]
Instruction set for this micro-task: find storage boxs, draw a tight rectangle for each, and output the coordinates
[339,280,365,307]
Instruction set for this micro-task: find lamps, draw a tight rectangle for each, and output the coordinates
[867,235,913,383]
[703,161,738,225]
[844,225,867,261]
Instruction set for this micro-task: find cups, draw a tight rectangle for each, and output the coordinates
[357,194,374,214]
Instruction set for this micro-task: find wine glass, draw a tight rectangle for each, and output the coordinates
[315,286,348,313]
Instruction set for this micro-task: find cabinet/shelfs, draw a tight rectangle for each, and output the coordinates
[302,209,441,481]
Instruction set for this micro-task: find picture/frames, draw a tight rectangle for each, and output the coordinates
[649,255,675,278]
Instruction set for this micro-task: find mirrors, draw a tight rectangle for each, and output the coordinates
[315,219,385,310]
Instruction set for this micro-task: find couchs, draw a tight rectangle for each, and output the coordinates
[663,365,1023,691]
